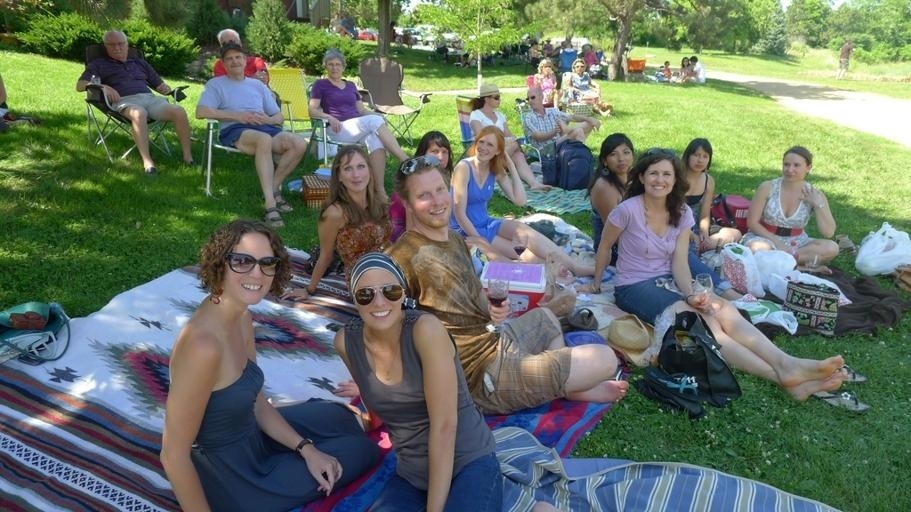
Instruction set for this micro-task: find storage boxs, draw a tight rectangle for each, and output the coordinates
[480,261,547,317]
[302,175,331,209]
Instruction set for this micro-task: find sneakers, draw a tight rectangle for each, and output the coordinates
[835,234,856,255]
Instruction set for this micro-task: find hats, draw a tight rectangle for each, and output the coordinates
[478,83,502,97]
[598,312,660,363]
[220,43,243,62]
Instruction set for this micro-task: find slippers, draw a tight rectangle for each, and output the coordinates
[647,366,702,402]
[661,311,743,403]
[569,308,597,331]
[812,360,868,414]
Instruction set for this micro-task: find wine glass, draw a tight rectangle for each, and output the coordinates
[485,277,509,335]
[694,272,714,315]
[511,231,529,263]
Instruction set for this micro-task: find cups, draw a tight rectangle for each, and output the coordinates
[805,254,822,269]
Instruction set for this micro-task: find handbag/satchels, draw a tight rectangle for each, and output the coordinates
[893,262,911,292]
[784,280,840,336]
[541,153,557,184]
[0,298,73,367]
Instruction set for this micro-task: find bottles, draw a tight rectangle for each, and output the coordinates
[789,242,800,269]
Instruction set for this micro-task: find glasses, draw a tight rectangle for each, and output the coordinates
[490,95,500,100]
[225,251,277,276]
[402,155,443,173]
[647,148,673,156]
[526,95,536,100]
[353,283,406,306]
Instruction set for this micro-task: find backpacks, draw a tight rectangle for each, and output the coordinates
[556,139,593,190]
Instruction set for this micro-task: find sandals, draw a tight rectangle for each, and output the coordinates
[264,190,295,228]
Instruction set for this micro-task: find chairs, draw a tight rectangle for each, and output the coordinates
[457,41,606,171]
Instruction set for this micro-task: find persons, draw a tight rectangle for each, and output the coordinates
[660,55,704,85]
[76,29,410,229]
[397,29,469,68]
[0,78,9,115]
[469,38,614,191]
[160,126,849,511]
[836,40,856,80]
